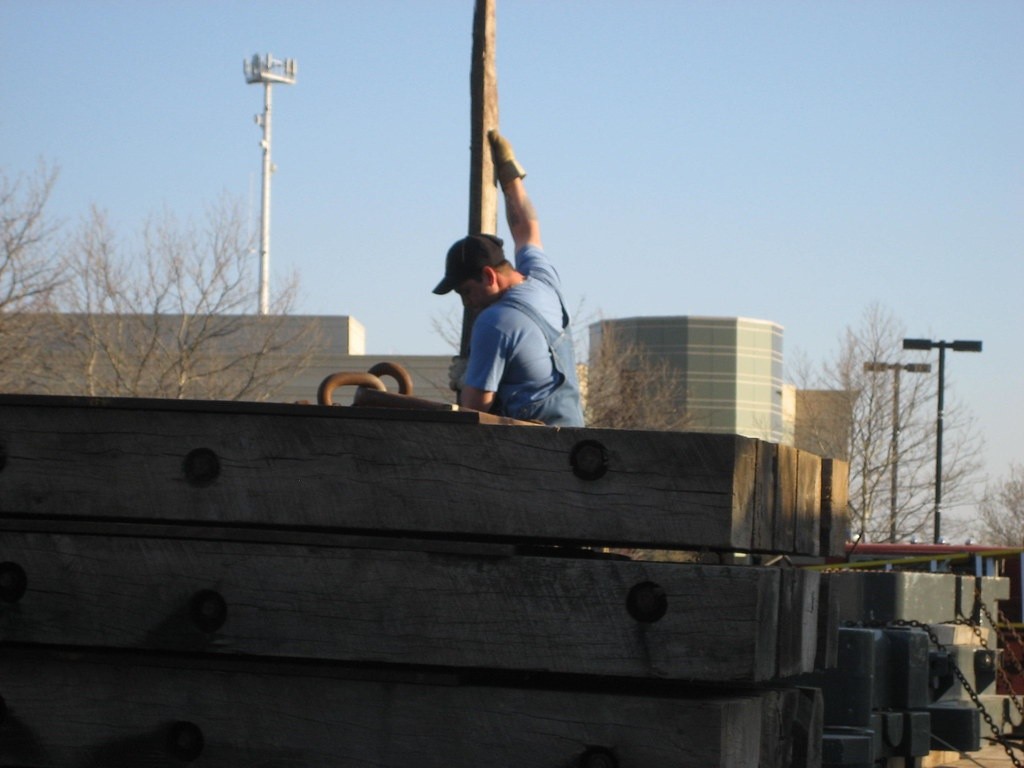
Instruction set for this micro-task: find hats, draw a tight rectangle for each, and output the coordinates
[432,234,506,295]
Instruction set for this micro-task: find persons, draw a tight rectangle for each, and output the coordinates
[432,130,585,428]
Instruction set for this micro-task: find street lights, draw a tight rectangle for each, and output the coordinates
[243,50,297,313]
[863,360,931,543]
[902,338,982,543]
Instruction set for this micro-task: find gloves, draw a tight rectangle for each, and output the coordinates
[448,355,471,392]
[470,130,525,186]
